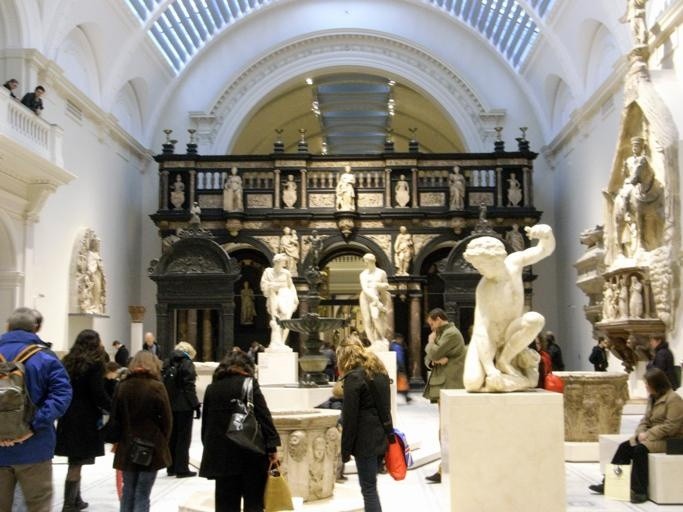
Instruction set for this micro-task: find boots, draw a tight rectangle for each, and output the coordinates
[62,477,88,512]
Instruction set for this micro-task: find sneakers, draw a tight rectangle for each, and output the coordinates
[589,484,604,492]
[631,488,647,502]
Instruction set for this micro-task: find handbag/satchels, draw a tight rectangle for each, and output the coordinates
[161,356,181,399]
[224,399,268,454]
[127,438,154,467]
[604,463,631,501]
[263,466,294,512]
[386,429,413,482]
[544,373,563,392]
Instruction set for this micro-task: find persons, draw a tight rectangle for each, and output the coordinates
[110,351,172,512]
[393,225,415,277]
[280,174,297,191]
[113,340,129,367]
[328,306,467,512]
[0,307,72,512]
[527,330,682,503]
[601,136,656,320]
[86,238,102,310]
[461,223,557,392]
[378,288,395,341]
[358,252,389,345]
[239,226,325,346]
[245,341,265,365]
[393,173,411,206]
[198,350,281,512]
[161,341,200,479]
[53,330,115,512]
[334,164,357,210]
[170,166,245,224]
[505,171,522,207]
[143,332,162,360]
[443,165,469,209]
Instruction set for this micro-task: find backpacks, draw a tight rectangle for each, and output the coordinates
[0,344,45,445]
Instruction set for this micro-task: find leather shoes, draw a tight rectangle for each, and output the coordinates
[168,470,196,477]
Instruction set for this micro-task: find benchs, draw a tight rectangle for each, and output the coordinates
[599,433,683,505]
